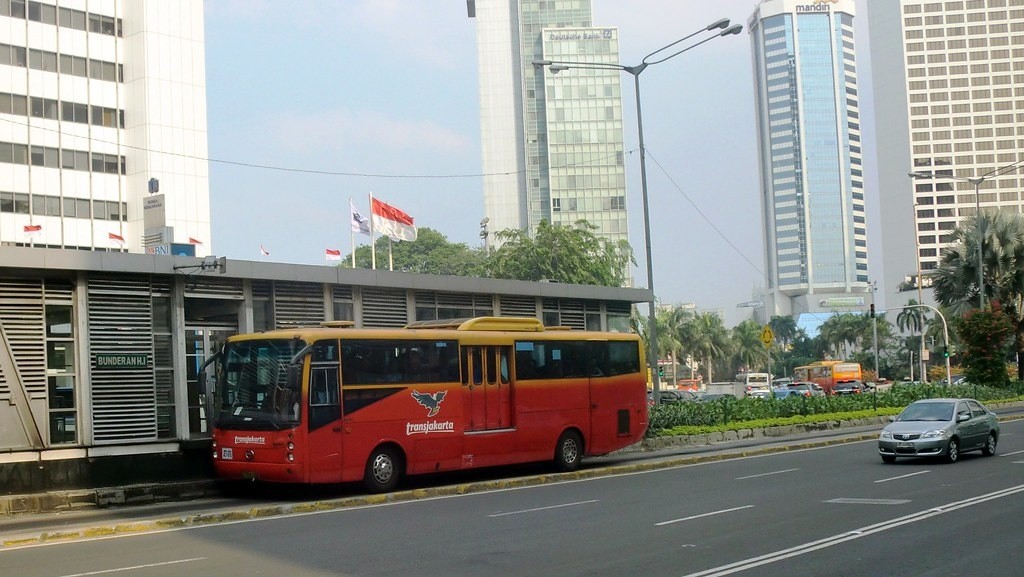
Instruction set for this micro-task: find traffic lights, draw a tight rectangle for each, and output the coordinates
[870,304,875,318]
[944,345,949,358]
[951,345,956,356]
[659,366,663,376]
[912,353,918,363]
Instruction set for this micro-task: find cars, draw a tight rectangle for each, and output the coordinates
[815,384,826,397]
[773,384,790,400]
[671,390,702,402]
[878,378,886,381]
[953,376,969,385]
[647,390,684,404]
[749,390,771,401]
[876,395,1000,461]
[941,376,961,384]
[902,376,911,380]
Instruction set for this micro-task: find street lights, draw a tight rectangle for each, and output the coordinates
[907,159,1024,309]
[867,280,880,382]
[531,18,742,400]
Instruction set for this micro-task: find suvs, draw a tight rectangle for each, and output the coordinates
[831,380,864,396]
[787,382,823,397]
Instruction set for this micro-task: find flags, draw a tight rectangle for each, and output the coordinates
[372,196,417,243]
[326,248,341,261]
[108,232,127,246]
[351,203,371,237]
[189,237,204,247]
[261,245,270,256]
[24,225,42,237]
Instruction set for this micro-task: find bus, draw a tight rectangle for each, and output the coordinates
[792,361,862,393]
[734,373,775,400]
[648,359,704,381]
[677,380,702,392]
[196,315,650,488]
